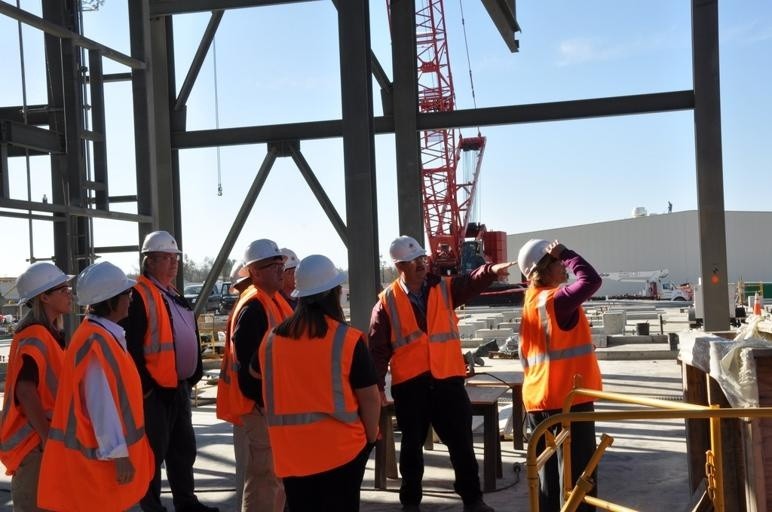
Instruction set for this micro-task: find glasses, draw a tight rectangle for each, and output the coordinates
[400,256,428,264]
[255,262,285,272]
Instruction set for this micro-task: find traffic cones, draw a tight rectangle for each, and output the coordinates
[752,293,761,316]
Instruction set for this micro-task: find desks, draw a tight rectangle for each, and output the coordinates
[423,371,524,450]
[375,385,509,493]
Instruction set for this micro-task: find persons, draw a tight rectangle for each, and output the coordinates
[126,231,219,512]
[0,263,76,512]
[366,234,517,512]
[249,254,380,512]
[667,201,673,213]
[517,238,603,512]
[216,238,314,512]
[35,261,156,512]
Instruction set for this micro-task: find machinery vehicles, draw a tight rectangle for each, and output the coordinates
[688,275,749,329]
[385,0,527,306]
[586,269,692,305]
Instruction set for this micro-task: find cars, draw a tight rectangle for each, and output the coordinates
[184,281,241,315]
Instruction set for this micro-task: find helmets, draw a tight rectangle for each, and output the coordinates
[228,259,251,288]
[290,254,348,298]
[389,235,426,264]
[243,238,288,271]
[279,248,300,271]
[518,238,550,279]
[15,230,183,306]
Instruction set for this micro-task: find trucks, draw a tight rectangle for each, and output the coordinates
[736,281,772,306]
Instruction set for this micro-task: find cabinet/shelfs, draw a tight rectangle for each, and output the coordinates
[681,331,772,511]
